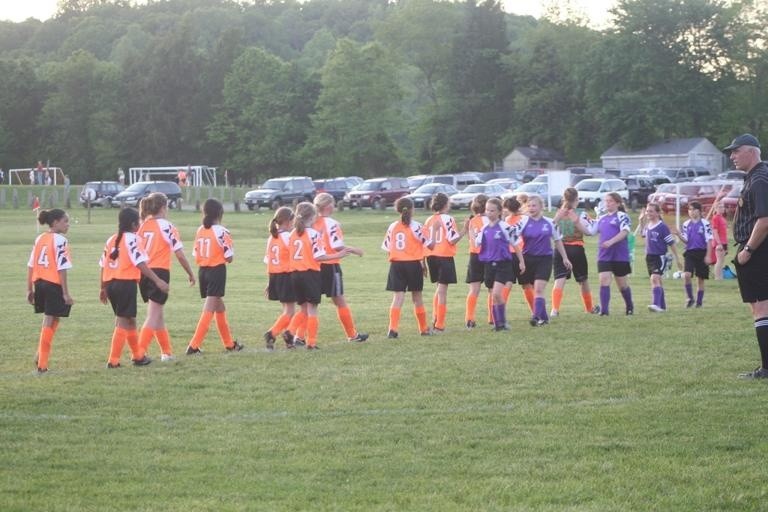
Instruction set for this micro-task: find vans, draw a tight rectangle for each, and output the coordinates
[241,177,314,209]
[112,182,180,210]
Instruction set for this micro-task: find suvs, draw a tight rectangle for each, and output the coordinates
[80,182,121,210]
[345,177,408,210]
[312,178,349,206]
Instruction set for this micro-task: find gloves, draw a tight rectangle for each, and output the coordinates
[716,244,723,250]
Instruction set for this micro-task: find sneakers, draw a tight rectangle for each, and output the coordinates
[264,329,369,351]
[584,305,600,314]
[184,345,203,357]
[736,366,768,381]
[432,320,444,332]
[387,329,398,340]
[418,326,431,336]
[34,359,52,374]
[225,341,246,353]
[107,354,178,369]
[624,309,633,316]
[685,300,702,308]
[549,308,559,319]
[466,316,548,331]
[601,312,609,317]
[647,303,666,313]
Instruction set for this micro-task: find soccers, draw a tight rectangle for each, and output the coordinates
[672,270,683,280]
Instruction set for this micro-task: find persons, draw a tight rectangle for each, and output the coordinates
[97,207,169,368]
[380,197,440,340]
[131,193,196,363]
[281,200,351,350]
[421,193,470,335]
[28,160,52,184]
[465,186,728,332]
[305,192,369,349]
[184,198,244,358]
[175,161,193,186]
[118,167,125,184]
[723,133,768,379]
[27,209,74,379]
[263,205,308,350]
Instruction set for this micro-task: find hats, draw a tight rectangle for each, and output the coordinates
[721,133,761,154]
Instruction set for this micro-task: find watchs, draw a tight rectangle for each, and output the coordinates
[743,244,754,254]
